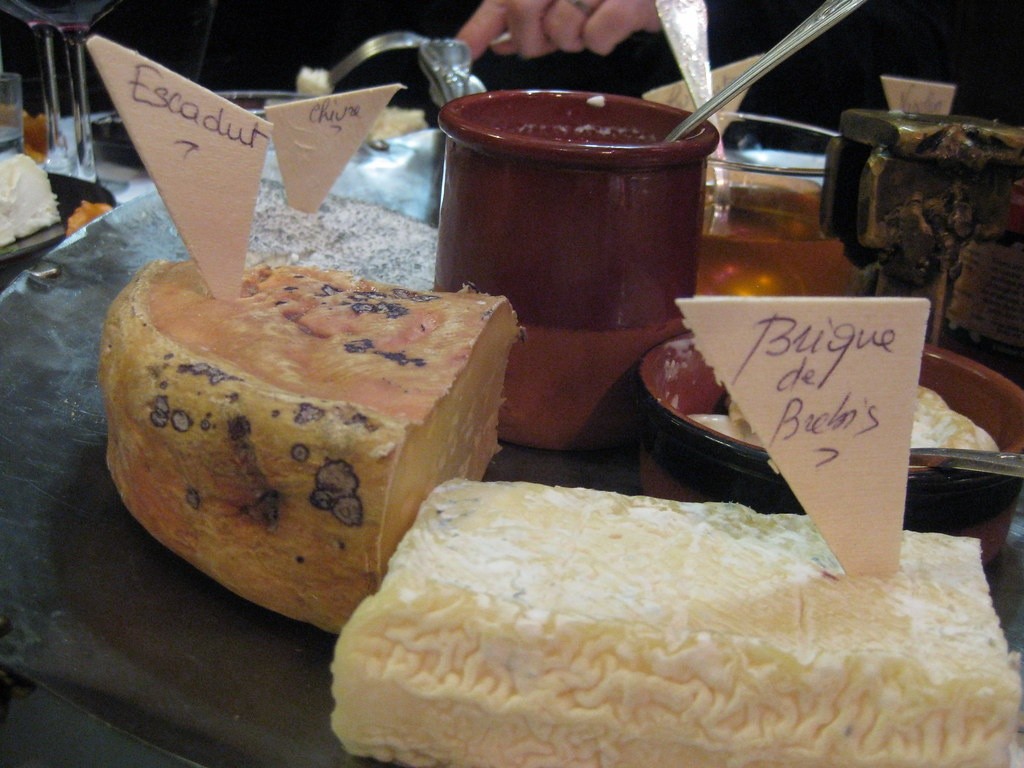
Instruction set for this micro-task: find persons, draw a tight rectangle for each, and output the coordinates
[452,0,662,57]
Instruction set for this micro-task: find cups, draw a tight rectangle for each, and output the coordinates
[438,89,720,452]
[694,111,861,298]
[0,73,24,163]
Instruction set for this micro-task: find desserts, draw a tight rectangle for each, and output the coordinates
[329,477,1024,768]
[0,152,63,249]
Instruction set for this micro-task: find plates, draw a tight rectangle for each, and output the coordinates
[92,91,315,173]
[0,172,116,262]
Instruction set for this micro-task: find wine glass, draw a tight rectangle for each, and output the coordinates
[1,0,131,199]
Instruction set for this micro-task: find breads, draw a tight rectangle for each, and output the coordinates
[96,260,519,635]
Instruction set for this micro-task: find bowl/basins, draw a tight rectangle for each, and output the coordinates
[630,332,1024,568]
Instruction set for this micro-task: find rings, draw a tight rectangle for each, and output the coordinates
[566,0,591,16]
